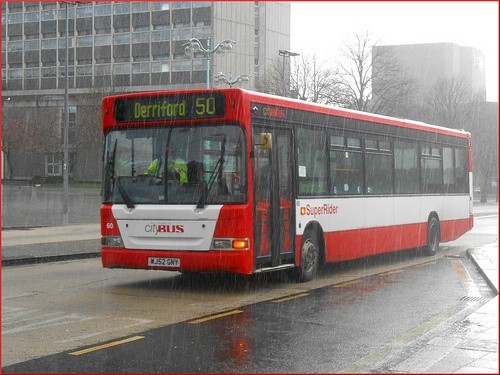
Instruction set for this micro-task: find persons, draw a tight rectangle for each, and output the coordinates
[145,140,188,185]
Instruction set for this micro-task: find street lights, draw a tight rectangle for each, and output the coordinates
[182,38,235,185]
[278,48,300,96]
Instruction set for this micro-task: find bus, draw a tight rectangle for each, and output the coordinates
[99,86,474,283]
